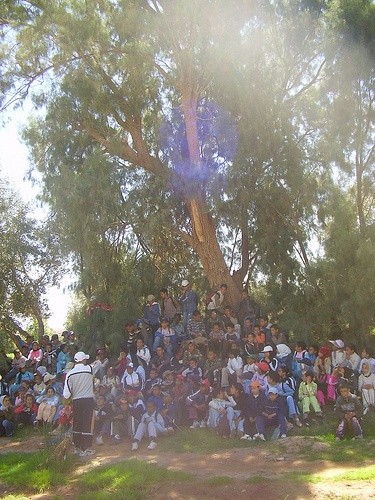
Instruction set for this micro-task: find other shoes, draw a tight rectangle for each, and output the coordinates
[303,416,310,426]
[294,417,302,426]
[131,441,139,451]
[239,433,252,440]
[190,419,208,429]
[164,427,174,433]
[281,433,287,438]
[115,434,120,444]
[254,433,265,442]
[148,439,160,450]
[75,447,95,456]
[96,435,104,445]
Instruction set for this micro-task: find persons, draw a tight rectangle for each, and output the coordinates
[0,277,375,456]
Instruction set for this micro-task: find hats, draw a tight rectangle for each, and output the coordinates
[247,354,257,360]
[249,381,260,387]
[261,346,273,353]
[200,380,210,387]
[74,351,90,362]
[128,362,135,368]
[297,358,311,366]
[339,361,352,370]
[44,374,56,382]
[268,388,278,394]
[329,339,344,349]
[255,363,268,373]
[37,366,46,374]
